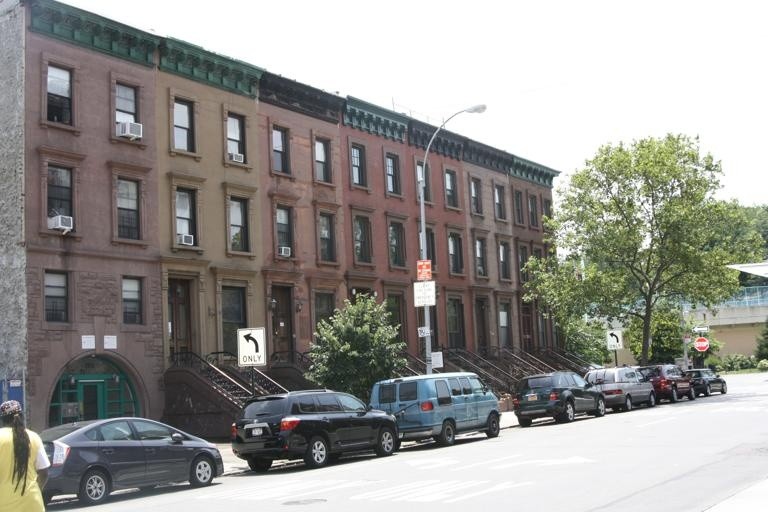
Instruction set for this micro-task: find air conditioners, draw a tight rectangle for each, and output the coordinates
[116,121,144,139]
[177,234,194,246]
[52,215,72,232]
[278,246,290,256]
[230,153,244,163]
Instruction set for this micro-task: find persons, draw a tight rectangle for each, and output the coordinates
[0,400,51,512]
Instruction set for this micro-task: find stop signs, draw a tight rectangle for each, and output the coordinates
[693,337,709,352]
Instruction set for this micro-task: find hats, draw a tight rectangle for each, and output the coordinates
[0,400,22,417]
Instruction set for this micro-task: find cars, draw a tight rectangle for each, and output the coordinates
[584,364,727,412]
[231,389,400,471]
[36,417,224,506]
[512,370,605,427]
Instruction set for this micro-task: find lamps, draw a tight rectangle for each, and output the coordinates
[270,299,276,308]
[297,302,303,311]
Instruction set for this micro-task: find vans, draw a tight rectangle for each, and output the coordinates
[370,371,502,446]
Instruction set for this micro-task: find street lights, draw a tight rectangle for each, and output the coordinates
[418,103,487,374]
